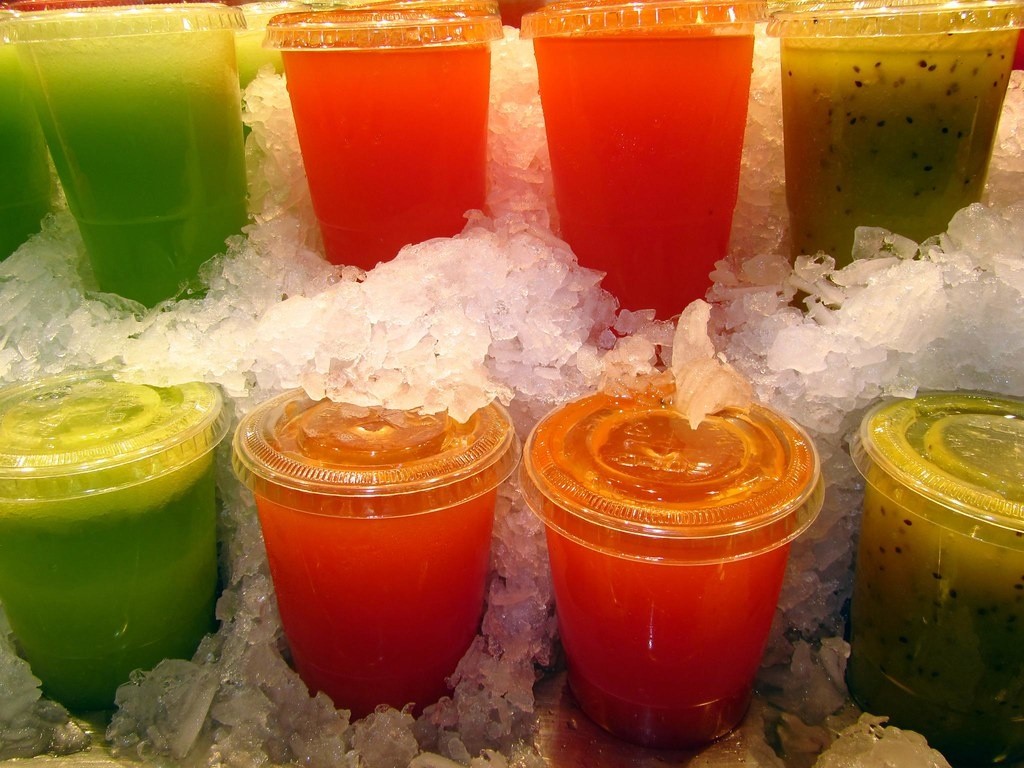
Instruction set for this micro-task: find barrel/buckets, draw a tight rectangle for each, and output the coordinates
[0,367,230,714]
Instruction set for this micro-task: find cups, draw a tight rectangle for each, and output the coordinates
[232,0,313,149]
[1010,28,1024,70]
[520,0,757,367]
[0,9,57,264]
[517,388,827,750]
[260,0,504,282]
[4,3,248,309]
[498,0,545,29]
[842,389,1024,768]
[767,0,1024,312]
[232,387,524,726]
